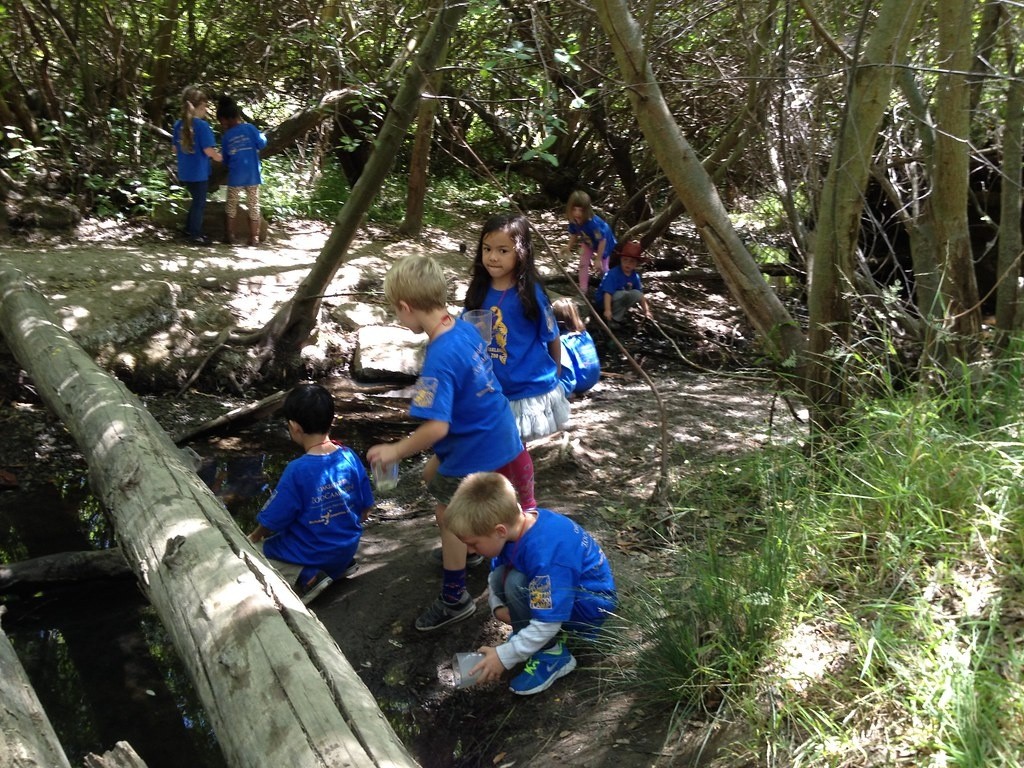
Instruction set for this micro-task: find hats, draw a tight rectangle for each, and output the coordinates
[616,241,645,259]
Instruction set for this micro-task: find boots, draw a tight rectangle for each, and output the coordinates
[223,214,235,244]
[247,219,261,246]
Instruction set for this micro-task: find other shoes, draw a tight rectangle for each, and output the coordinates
[343,559,361,576]
[188,236,212,246]
[296,573,333,605]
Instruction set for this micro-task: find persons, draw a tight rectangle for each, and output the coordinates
[366,256,520,631]
[463,213,571,447]
[218,96,268,247]
[248,385,373,606]
[566,191,617,299]
[551,298,600,399]
[443,472,618,695]
[172,84,222,247]
[596,239,653,328]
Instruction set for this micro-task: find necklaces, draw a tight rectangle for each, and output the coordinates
[305,439,346,453]
[482,275,511,321]
[503,514,529,588]
[429,312,451,342]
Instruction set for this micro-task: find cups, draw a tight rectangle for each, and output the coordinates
[370,460,399,492]
[452,651,487,689]
[463,310,492,346]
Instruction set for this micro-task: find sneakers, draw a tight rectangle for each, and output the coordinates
[415,590,476,631]
[509,633,576,695]
[432,548,483,566]
[469,575,489,603]
